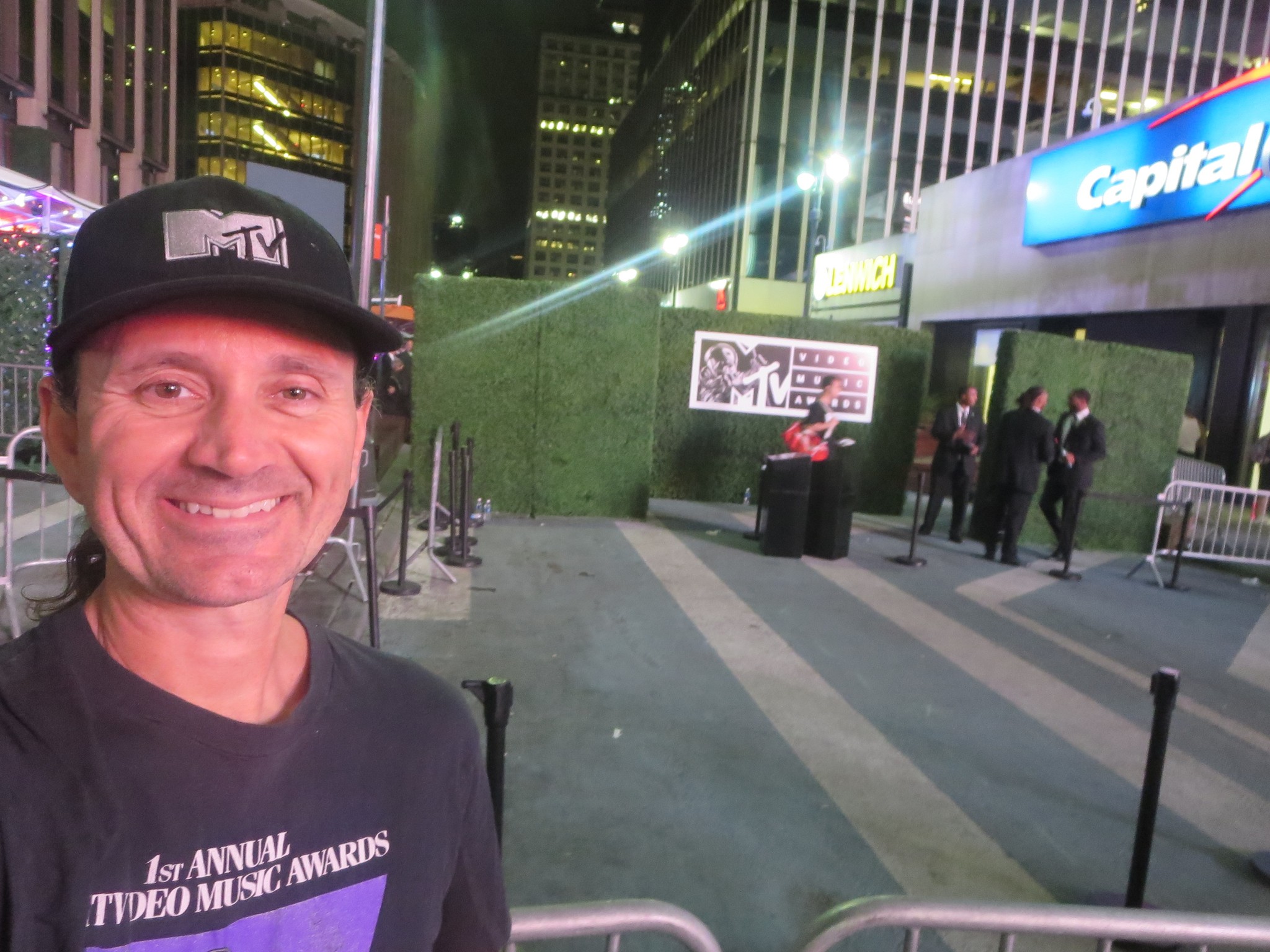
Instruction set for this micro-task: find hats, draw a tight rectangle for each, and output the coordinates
[44,175,407,355]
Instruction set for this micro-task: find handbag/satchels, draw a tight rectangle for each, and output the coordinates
[783,421,829,463]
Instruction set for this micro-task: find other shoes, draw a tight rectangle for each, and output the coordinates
[983,551,995,561]
[949,534,964,544]
[1001,556,1022,567]
[1051,546,1067,561]
[919,524,932,535]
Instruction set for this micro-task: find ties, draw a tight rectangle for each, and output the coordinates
[961,411,966,427]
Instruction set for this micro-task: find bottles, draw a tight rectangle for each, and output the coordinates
[476,498,483,518]
[484,500,490,520]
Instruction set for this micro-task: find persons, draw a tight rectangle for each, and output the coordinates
[804,374,841,442]
[0,177,514,952]
[1250,433,1270,491]
[917,385,987,543]
[371,339,413,414]
[982,386,1054,565]
[1038,388,1106,562]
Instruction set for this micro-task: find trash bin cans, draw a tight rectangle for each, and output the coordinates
[761,451,812,558]
[805,438,857,560]
